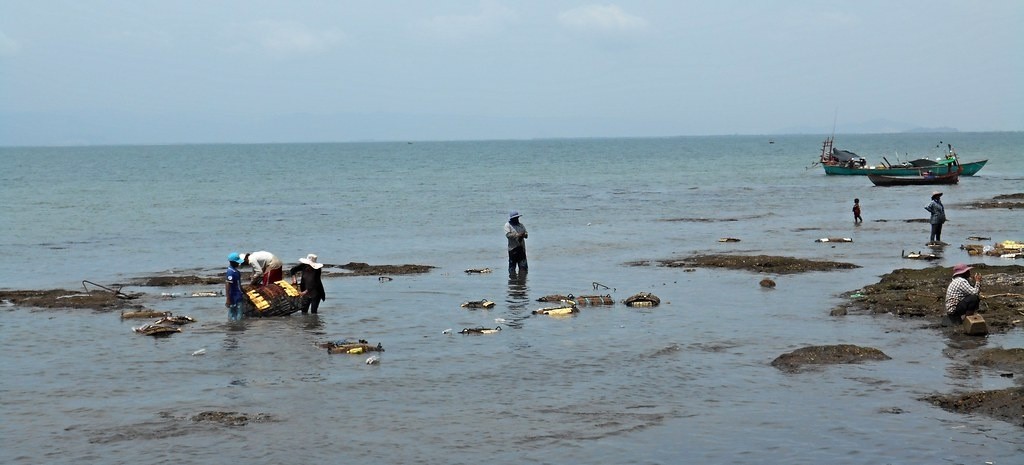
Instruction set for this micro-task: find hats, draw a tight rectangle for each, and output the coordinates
[510,211,522,220]
[931,191,943,200]
[299,254,324,269]
[239,253,250,265]
[953,264,974,276]
[228,253,244,263]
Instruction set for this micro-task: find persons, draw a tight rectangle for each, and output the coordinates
[504,212,528,272]
[239,252,282,286]
[945,263,982,316]
[290,254,325,314]
[852,198,862,223]
[924,191,946,241]
[225,252,245,321]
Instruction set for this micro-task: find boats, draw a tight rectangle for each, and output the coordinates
[867,172,959,187]
[819,135,988,177]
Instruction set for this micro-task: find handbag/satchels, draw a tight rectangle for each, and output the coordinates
[938,214,945,224]
